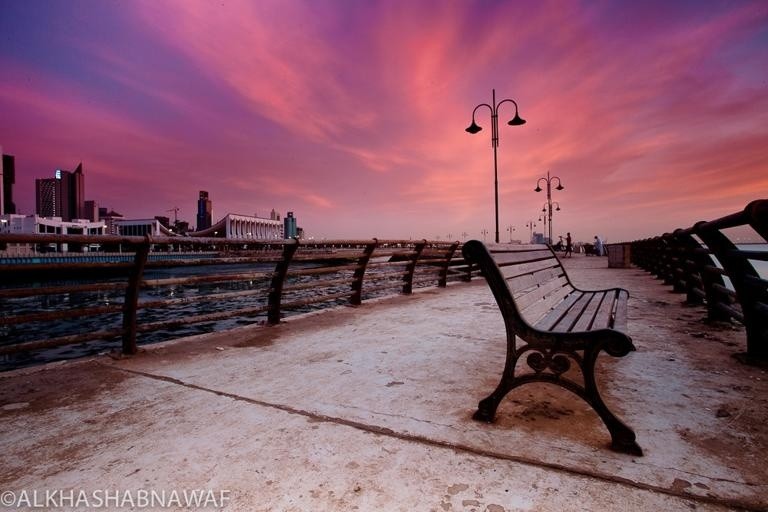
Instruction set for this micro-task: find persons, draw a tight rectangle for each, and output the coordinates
[558,232,572,258]
[592,236,603,256]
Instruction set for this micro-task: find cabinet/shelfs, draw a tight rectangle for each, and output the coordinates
[607,244,632,268]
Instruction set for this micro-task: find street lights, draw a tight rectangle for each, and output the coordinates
[436,171,564,242]
[465,87,526,243]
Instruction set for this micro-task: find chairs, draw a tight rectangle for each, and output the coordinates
[583,245,600,256]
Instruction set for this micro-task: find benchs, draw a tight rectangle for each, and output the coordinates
[462,240,643,457]
